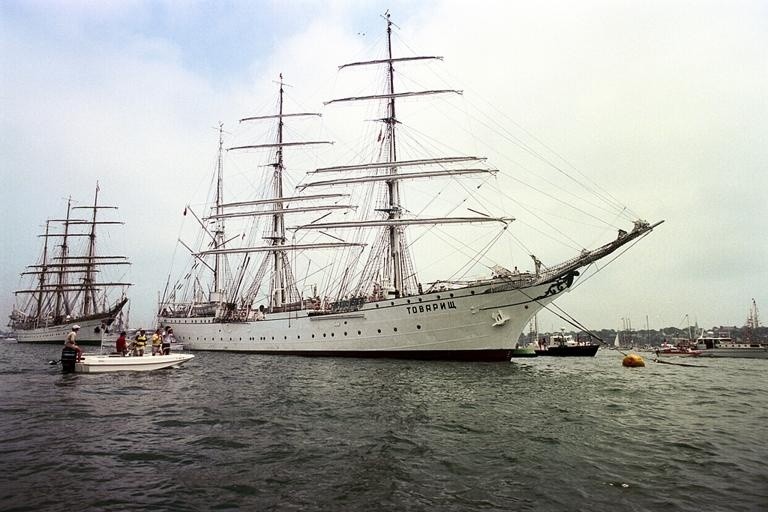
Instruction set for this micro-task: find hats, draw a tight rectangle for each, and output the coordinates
[73,325,80,329]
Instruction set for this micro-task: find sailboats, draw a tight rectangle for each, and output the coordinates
[7,179,134,345]
[152,7,664,360]
[581,315,699,358]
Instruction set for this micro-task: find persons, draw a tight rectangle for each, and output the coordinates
[116,331,129,357]
[65,324,84,363]
[134,330,148,356]
[541,338,546,348]
[151,329,164,355]
[538,338,541,349]
[512,266,520,274]
[162,326,175,355]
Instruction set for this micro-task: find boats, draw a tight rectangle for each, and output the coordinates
[75,352,194,374]
[513,346,537,358]
[692,337,767,361]
[97,331,186,357]
[534,328,598,358]
[5,338,17,344]
